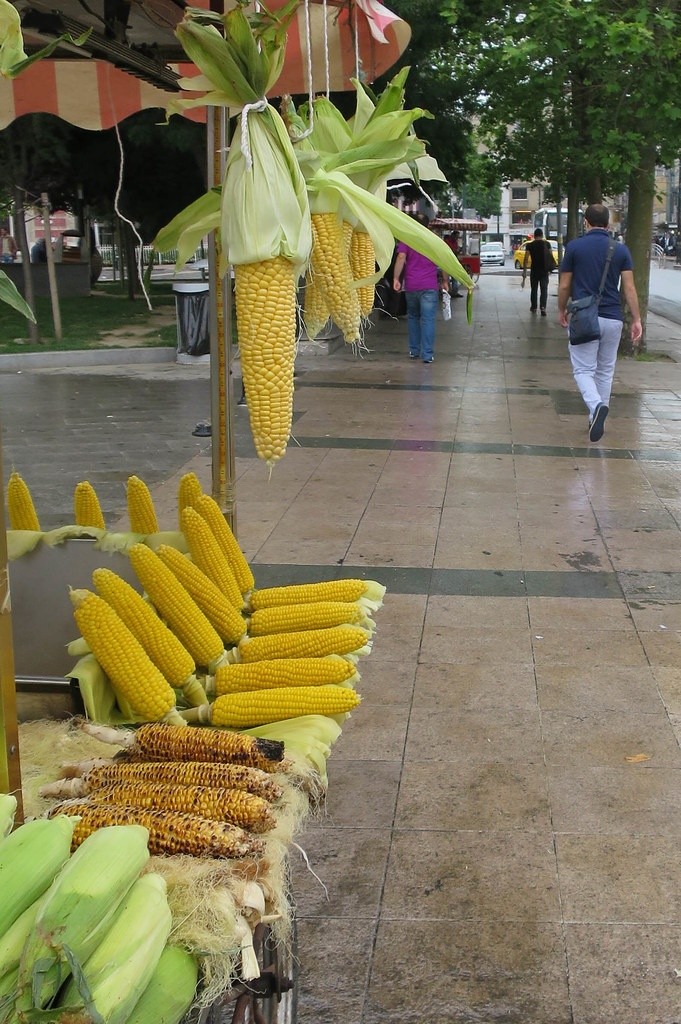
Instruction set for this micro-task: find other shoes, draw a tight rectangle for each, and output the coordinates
[541,307,546,316]
[530,308,536,312]
[588,402,609,442]
[424,357,434,363]
[409,351,419,358]
[452,293,463,298]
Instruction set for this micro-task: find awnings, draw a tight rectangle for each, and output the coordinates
[429,217,486,229]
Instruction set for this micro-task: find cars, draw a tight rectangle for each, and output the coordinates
[480,241,505,267]
[513,239,566,269]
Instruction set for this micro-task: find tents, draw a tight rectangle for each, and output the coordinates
[0,0,415,834]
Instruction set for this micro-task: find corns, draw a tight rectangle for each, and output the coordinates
[231,94,376,467]
[68,494,368,727]
[0,792,202,1024]
[7,469,203,534]
[34,722,293,858]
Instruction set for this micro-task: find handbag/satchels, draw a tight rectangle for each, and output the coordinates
[543,241,556,272]
[565,295,601,345]
[442,289,451,321]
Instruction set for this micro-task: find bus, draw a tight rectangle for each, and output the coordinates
[531,208,583,246]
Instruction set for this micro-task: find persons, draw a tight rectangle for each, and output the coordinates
[443,231,463,298]
[467,233,481,256]
[394,214,449,362]
[0,227,18,263]
[557,204,643,443]
[522,229,552,315]
